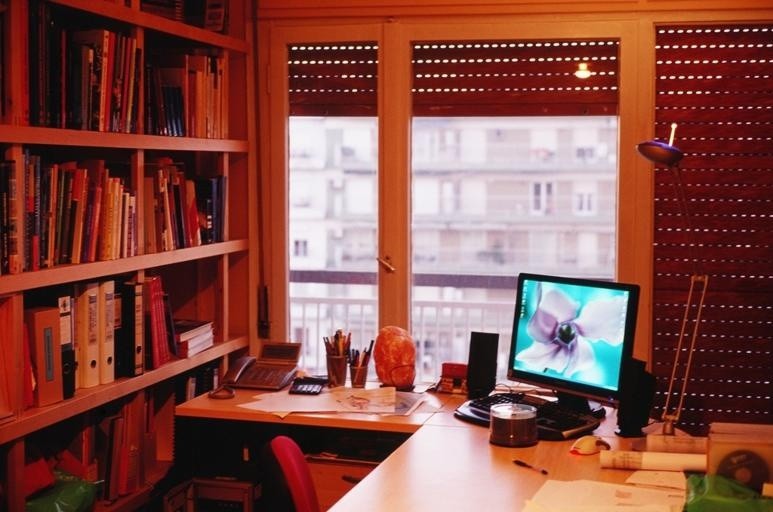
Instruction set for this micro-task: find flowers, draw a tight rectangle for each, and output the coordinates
[514,287,627,378]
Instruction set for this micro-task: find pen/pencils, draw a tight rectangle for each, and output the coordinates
[513,458,548,475]
[322,328,374,386]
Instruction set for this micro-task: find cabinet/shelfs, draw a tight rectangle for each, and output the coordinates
[0,0,256,512]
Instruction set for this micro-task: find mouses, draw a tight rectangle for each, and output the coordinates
[569,435,611,456]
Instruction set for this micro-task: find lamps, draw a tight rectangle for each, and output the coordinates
[629,121,712,439]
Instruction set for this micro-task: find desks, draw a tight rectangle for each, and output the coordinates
[172,373,689,512]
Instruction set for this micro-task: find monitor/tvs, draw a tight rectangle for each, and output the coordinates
[506,272,640,416]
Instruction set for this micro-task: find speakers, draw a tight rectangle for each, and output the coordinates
[466,330,501,398]
[614,358,657,439]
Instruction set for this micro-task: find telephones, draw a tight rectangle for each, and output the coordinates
[221,341,302,390]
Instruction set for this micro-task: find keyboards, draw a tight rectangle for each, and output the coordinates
[455,391,601,440]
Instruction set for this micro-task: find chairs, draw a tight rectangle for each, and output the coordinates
[301,450,386,512]
[267,435,324,512]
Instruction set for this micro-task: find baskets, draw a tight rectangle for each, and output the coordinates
[327,356,347,387]
[350,367,367,388]
